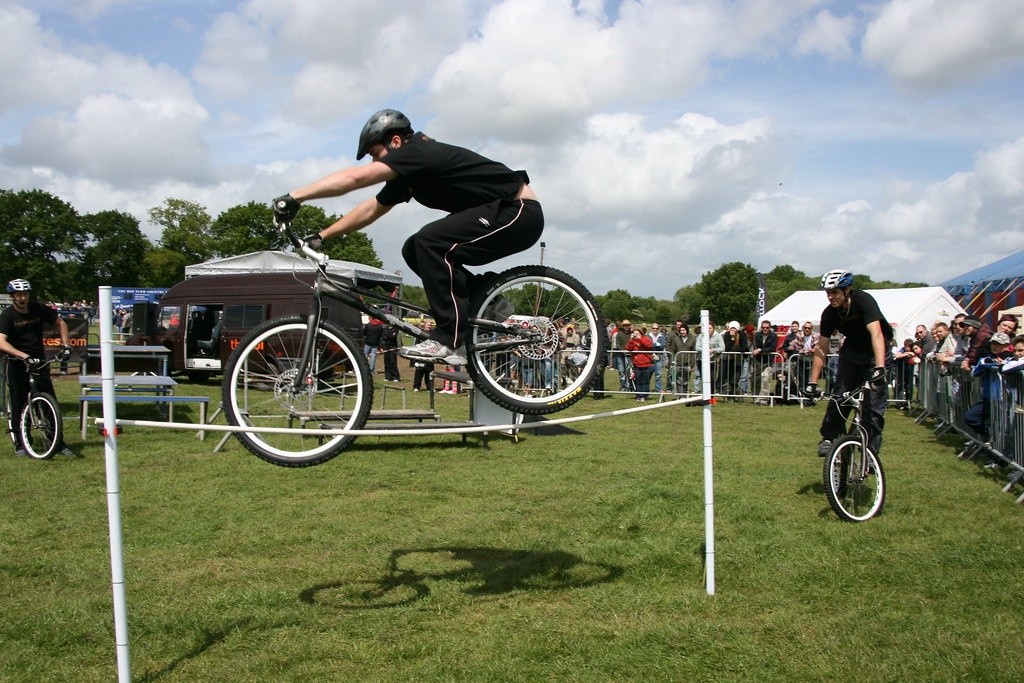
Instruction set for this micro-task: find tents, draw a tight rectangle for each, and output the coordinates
[758,286,969,375]
[184,247,403,357]
[932,249,1024,332]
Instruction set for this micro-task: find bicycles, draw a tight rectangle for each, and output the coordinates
[798,374,887,524]
[6,354,64,460]
[221,200,609,468]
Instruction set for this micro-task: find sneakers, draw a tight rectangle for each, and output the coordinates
[817,439,832,456]
[487,297,514,323]
[399,334,469,366]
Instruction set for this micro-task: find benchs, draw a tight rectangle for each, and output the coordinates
[80,395,210,441]
[288,409,442,439]
[317,422,489,449]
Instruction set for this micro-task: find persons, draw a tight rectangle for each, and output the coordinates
[964,313,1024,438]
[271,108,544,366]
[480,317,582,397]
[59,306,70,374]
[741,321,915,409]
[413,319,436,393]
[806,269,895,472]
[895,313,993,435]
[112,306,131,341]
[43,300,96,328]
[585,319,749,407]
[362,315,401,383]
[438,365,458,394]
[0,280,78,458]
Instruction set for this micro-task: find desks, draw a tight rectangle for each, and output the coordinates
[428,370,519,421]
[79,376,178,422]
[82,346,172,418]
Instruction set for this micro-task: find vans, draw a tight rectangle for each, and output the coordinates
[124,273,365,382]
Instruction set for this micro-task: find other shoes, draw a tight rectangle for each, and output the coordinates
[197,339,214,350]
[57,447,73,457]
[439,389,457,394]
[15,448,26,457]
[384,378,389,383]
[393,379,399,382]
[965,440,976,446]
[413,388,418,393]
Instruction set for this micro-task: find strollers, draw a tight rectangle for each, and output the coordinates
[619,351,636,391]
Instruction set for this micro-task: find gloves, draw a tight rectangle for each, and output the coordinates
[871,368,887,387]
[25,356,40,367]
[273,194,299,223]
[806,382,821,402]
[58,346,71,361]
[303,233,323,250]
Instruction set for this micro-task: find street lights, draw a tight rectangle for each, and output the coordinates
[534,242,545,315]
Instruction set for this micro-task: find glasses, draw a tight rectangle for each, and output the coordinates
[624,323,631,327]
[652,327,659,330]
[916,329,925,335]
[804,326,812,330]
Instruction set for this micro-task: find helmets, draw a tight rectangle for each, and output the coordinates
[356,109,412,161]
[6,279,32,294]
[820,269,854,290]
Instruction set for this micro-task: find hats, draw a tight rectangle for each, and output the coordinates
[729,321,740,331]
[959,319,982,329]
[621,320,632,326]
[988,333,1011,344]
